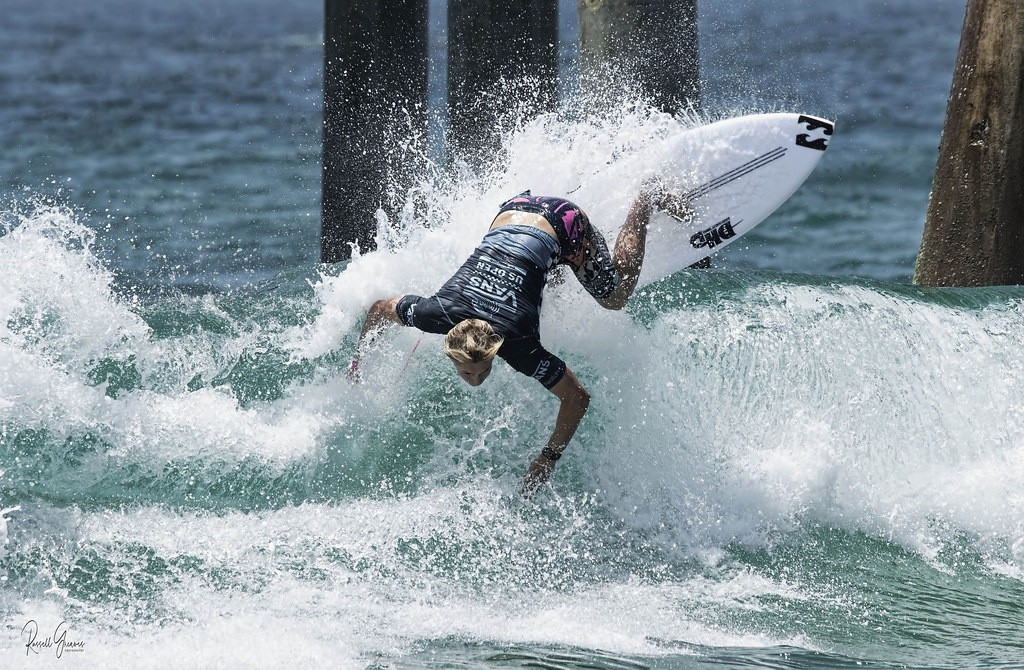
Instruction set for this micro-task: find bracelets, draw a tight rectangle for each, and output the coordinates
[541,444,563,461]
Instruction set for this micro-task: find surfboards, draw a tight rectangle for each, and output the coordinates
[576,114,835,288]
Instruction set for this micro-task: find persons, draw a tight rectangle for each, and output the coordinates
[345,174,698,501]
